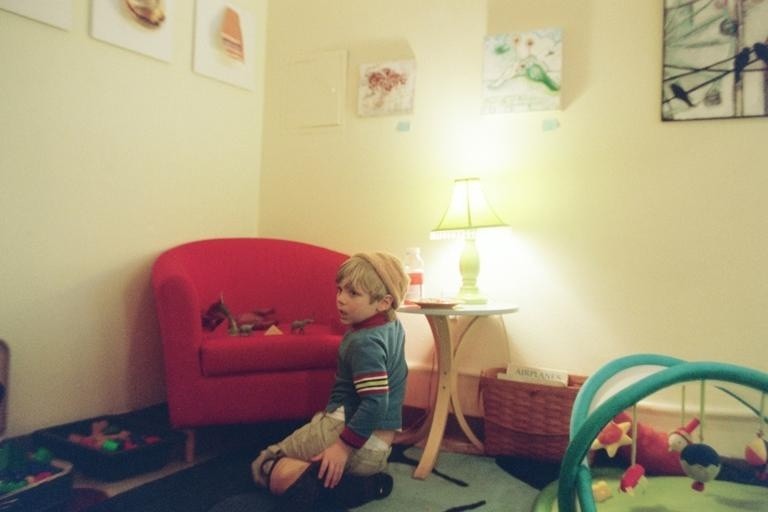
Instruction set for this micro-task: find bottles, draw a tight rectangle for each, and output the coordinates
[403,247,424,305]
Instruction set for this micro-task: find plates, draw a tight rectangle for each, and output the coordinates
[413,297,462,310]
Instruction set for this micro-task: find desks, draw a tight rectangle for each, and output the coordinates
[391,301,520,481]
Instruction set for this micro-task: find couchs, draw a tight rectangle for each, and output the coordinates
[148,237,352,461]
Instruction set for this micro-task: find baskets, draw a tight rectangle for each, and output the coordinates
[33,420,168,482]
[474,367,597,472]
[1,451,74,511]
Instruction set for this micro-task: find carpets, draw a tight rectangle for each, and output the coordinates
[1,404,767,511]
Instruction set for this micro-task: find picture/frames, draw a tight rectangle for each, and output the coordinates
[0,1,75,31]
[284,47,347,128]
[661,2,767,123]
[90,0,179,64]
[192,0,255,90]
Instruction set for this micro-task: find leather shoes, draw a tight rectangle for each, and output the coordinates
[339,473,394,508]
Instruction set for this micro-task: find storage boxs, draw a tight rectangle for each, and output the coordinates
[1,438,78,510]
[33,414,186,481]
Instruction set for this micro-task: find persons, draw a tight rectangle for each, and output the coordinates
[248,247,411,498]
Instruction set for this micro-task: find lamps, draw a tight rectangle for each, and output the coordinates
[427,174,513,305]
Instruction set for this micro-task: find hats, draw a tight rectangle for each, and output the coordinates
[352,250,411,311]
